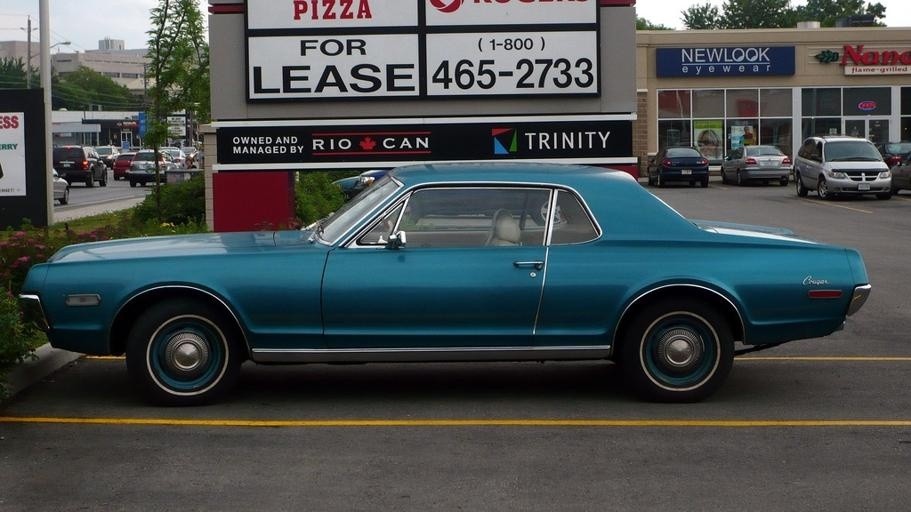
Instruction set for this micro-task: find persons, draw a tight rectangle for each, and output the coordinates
[737,127,759,146]
[699,128,721,157]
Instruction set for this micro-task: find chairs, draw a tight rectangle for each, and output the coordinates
[486,208,524,247]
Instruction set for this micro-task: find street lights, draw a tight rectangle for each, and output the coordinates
[23,17,72,88]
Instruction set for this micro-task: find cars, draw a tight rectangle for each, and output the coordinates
[325,167,388,202]
[9,156,875,410]
[645,146,711,190]
[51,141,205,205]
[791,133,911,201]
[718,142,793,188]
